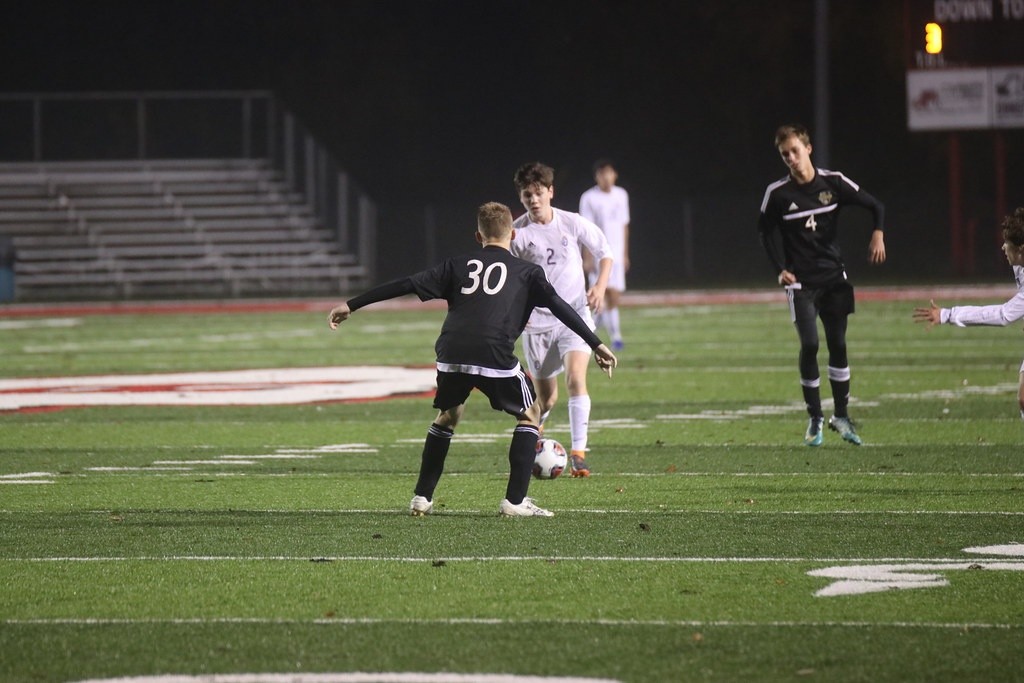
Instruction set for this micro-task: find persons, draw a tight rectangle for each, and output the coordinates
[579,159,630,351]
[330,202,618,516]
[912,209,1024,420]
[758,125,886,447]
[506,162,612,478]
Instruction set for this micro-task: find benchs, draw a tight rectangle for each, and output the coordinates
[0,156,370,301]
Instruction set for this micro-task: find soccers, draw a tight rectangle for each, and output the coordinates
[531,439,568,480]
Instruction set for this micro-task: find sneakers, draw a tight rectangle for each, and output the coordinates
[804,413,825,448]
[497,496,556,519]
[405,496,437,518]
[566,453,592,479]
[828,413,863,446]
